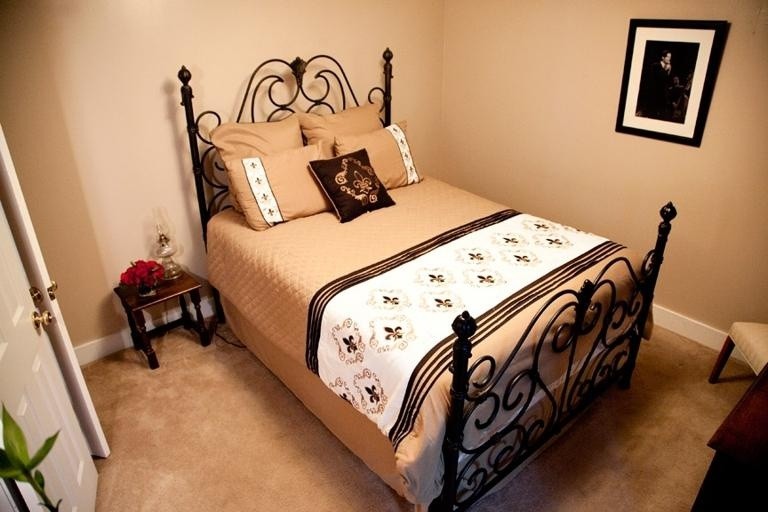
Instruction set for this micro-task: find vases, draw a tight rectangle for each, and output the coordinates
[138,284,157,297]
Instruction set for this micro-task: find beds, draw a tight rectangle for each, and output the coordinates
[178,47,677,511]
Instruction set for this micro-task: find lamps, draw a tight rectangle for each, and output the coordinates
[152,206,183,280]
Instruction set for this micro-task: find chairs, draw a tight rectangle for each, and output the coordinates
[708,321,768,384]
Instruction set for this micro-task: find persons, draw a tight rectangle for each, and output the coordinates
[642,47,684,122]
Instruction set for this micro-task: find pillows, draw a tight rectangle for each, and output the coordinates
[209,100,424,231]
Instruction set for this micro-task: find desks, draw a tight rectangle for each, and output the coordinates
[113,270,209,370]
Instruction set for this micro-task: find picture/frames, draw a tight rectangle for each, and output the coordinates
[615,18,730,147]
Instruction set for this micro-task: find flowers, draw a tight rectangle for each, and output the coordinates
[120,259,165,290]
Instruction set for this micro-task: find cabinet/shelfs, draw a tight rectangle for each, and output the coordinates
[690,362,768,512]
